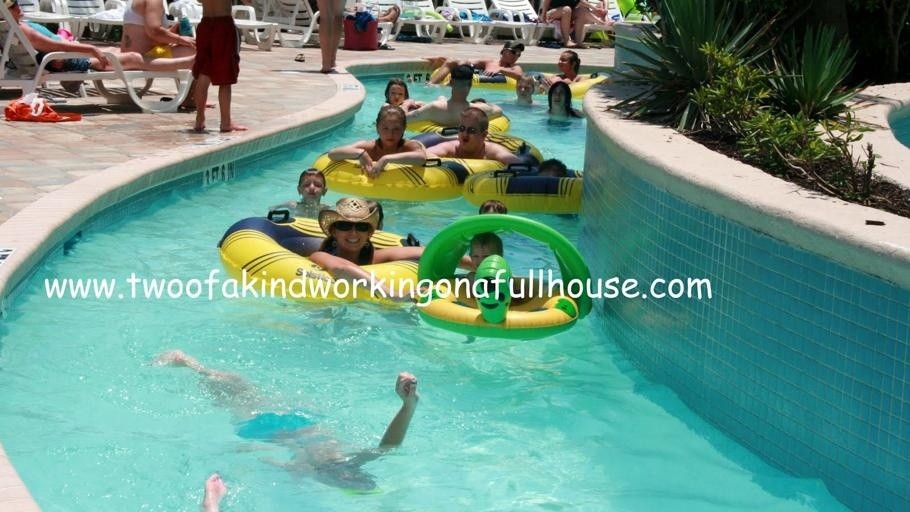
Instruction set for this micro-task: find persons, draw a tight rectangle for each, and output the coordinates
[315,1,614,180]
[155,351,420,496]
[204,473,225,511]
[462,233,540,297]
[308,197,426,299]
[479,199,507,215]
[120,0,197,58]
[0,1,196,70]
[192,0,250,132]
[270,168,331,219]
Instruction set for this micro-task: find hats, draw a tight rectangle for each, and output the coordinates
[319,197,379,237]
[502,41,525,55]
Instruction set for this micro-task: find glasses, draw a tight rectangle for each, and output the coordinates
[332,221,370,233]
[503,47,521,57]
[458,125,483,134]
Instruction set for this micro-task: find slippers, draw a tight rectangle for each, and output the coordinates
[381,44,394,50]
[295,53,304,61]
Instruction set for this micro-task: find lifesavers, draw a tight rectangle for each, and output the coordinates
[461,161,583,213]
[417,214,592,339]
[309,128,544,201]
[430,66,517,91]
[403,114,510,138]
[530,70,610,97]
[217,209,417,307]
